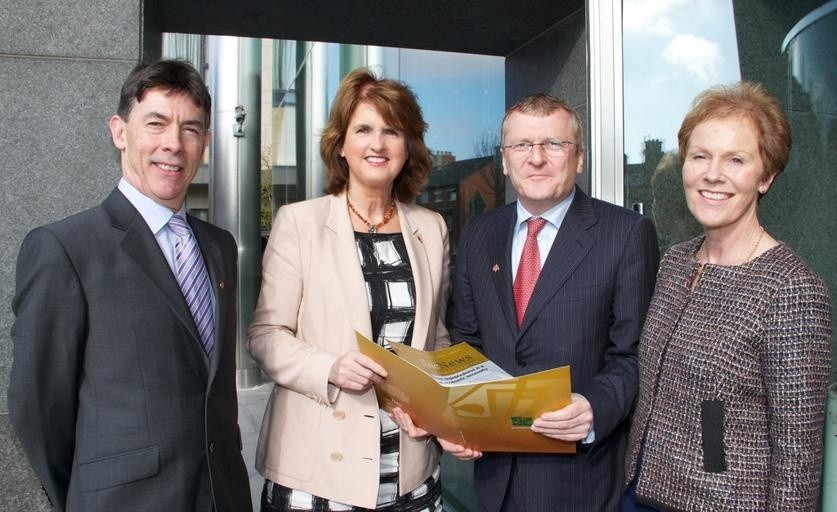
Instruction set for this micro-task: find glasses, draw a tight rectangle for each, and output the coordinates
[502,139,582,159]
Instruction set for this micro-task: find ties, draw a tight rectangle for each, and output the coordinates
[513,216,546,329]
[167,213,216,358]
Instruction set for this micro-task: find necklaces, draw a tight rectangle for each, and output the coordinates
[702,226,764,264]
[347,198,395,235]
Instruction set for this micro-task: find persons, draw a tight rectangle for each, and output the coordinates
[246,66,451,512]
[6,60,253,512]
[622,83,831,512]
[436,94,660,512]
